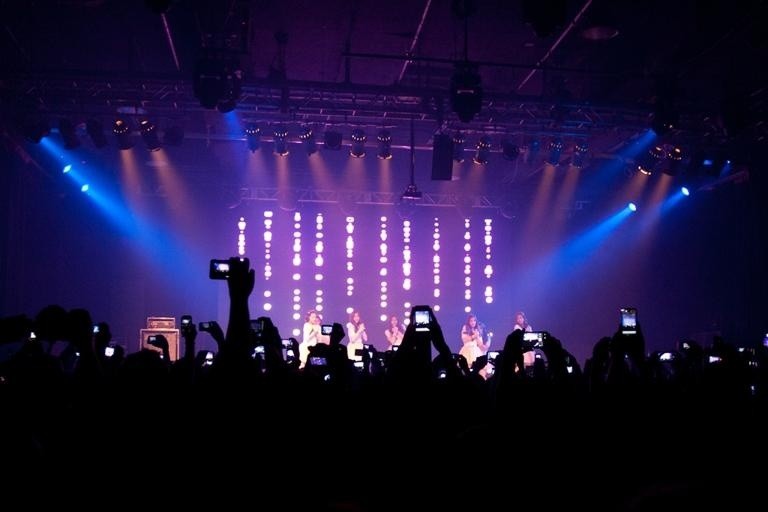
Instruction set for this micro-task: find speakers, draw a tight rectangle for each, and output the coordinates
[431,134,453,180]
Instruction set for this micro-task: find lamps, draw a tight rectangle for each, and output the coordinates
[636,140,685,177]
[23,111,162,154]
[398,118,424,201]
[192,72,241,114]
[243,122,395,163]
[452,127,589,170]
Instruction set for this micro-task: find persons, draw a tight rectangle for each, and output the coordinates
[0,255,767,511]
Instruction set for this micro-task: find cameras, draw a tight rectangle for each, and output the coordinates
[209,259,244,280]
[321,325,345,337]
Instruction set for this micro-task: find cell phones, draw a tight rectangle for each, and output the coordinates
[204,351,213,366]
[440,373,446,378]
[683,342,690,350]
[31,332,37,339]
[363,344,370,349]
[487,351,500,360]
[181,315,192,337]
[249,320,264,333]
[147,336,157,344]
[355,349,365,356]
[104,347,115,357]
[620,307,637,335]
[254,344,265,354]
[392,345,398,351]
[535,354,542,359]
[76,353,81,357]
[415,305,430,333]
[92,325,100,334]
[762,334,768,347]
[523,331,548,348]
[708,356,722,364]
[566,366,573,374]
[199,322,214,331]
[739,348,745,352]
[287,350,294,364]
[309,356,327,366]
[657,351,676,361]
[281,339,293,348]
